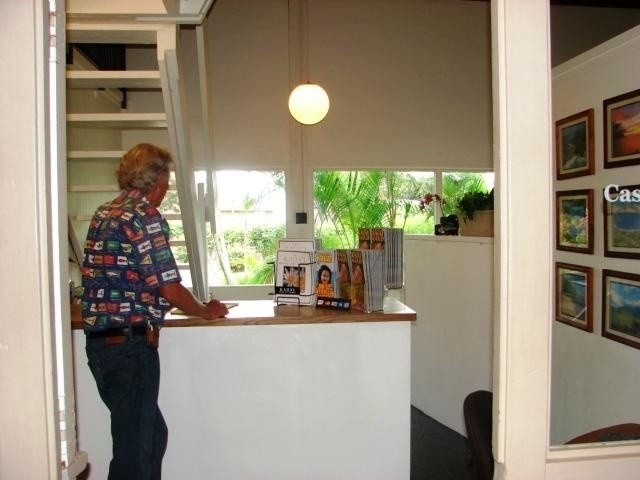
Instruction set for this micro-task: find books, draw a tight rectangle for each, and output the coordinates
[171,303,239,316]
[274,224,404,314]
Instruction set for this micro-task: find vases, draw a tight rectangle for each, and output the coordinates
[440,221,459,236]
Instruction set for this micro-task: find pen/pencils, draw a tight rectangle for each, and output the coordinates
[203,302,226,319]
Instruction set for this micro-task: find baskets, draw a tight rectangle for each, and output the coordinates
[458,209,493,237]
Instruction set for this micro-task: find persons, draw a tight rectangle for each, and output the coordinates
[81,142,229,480]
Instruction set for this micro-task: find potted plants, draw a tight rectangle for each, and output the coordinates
[456,186,494,236]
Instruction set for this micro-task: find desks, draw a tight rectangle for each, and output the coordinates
[403,234,493,442]
[71,295,418,479]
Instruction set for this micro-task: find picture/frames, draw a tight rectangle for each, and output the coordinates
[555,261,593,333]
[602,88,640,169]
[556,189,594,255]
[602,185,640,259]
[601,268,640,350]
[555,108,594,181]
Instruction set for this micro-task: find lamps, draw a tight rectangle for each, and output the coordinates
[288,0,330,126]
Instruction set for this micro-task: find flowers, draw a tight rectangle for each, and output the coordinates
[420,190,459,223]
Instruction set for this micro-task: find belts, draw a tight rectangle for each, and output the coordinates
[86,326,146,339]
